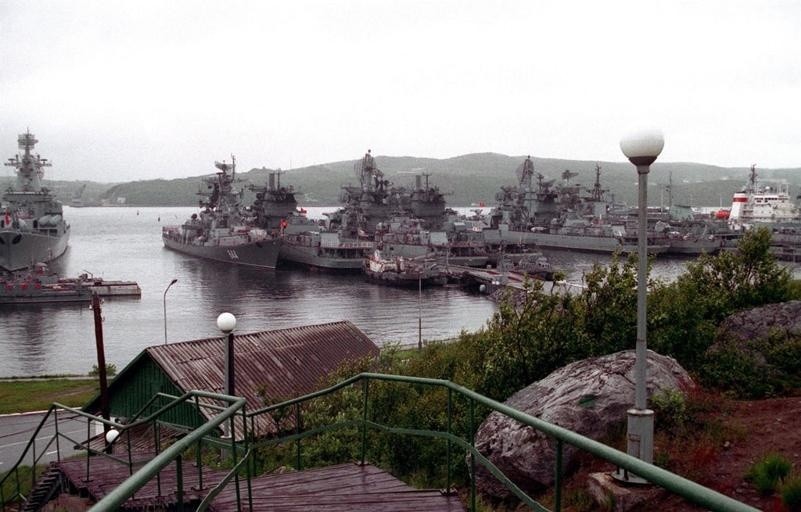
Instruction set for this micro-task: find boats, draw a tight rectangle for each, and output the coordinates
[0,125,70,273]
[0,280,144,306]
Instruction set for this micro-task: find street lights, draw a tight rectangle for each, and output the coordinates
[217,313,236,459]
[610,130,664,477]
[163,280,178,343]
